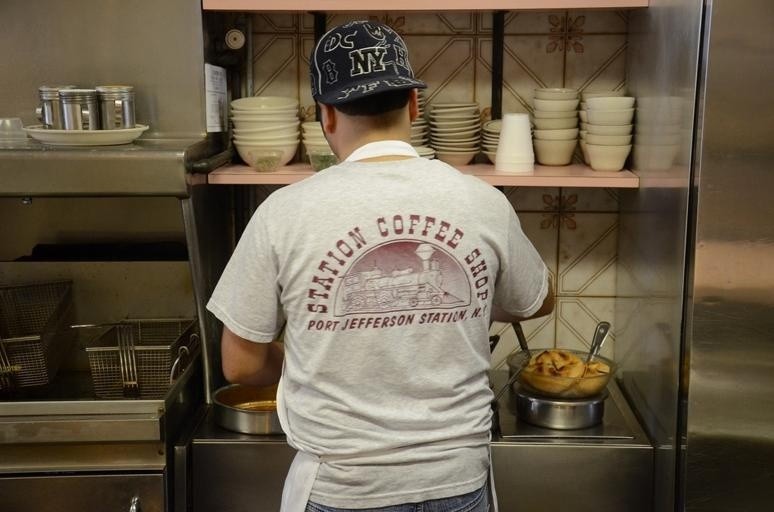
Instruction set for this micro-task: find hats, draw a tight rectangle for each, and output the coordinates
[310,20,428,105]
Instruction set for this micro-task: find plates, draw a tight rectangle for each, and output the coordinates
[22,125,149,147]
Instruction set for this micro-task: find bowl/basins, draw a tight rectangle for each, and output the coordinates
[302,120,340,172]
[532,87,580,167]
[230,95,302,171]
[210,383,288,436]
[481,118,534,165]
[401,86,435,161]
[580,87,634,172]
[429,103,482,168]
[506,348,617,397]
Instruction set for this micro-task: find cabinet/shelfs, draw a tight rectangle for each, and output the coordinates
[201,1,653,188]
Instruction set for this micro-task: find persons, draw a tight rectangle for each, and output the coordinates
[204,17,556,512]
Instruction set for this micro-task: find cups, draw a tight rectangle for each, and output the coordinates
[496,113,535,171]
[0,117,24,150]
[35,85,136,130]
